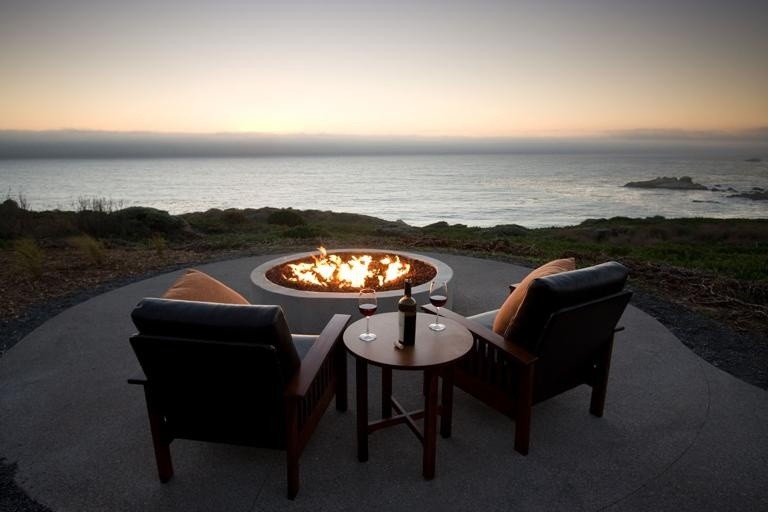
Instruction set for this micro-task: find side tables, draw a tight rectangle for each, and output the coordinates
[341,310,473,480]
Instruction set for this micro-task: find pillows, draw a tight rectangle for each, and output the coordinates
[492,256,576,335]
[163,268,249,304]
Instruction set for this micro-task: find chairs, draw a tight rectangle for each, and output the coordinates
[130,295,352,502]
[440,261,634,458]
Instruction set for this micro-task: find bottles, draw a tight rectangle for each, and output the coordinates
[397,279,417,346]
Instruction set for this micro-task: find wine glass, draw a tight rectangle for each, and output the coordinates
[428,280,448,331]
[358,289,378,342]
